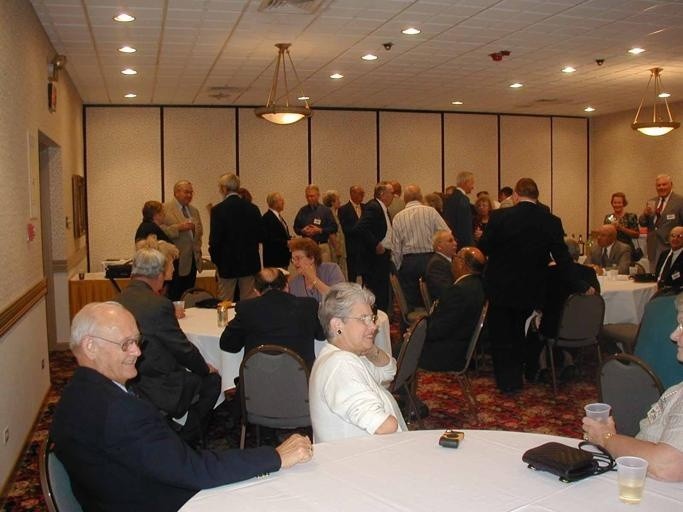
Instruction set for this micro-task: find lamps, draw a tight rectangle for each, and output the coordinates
[629,68,680,137]
[254,42,312,126]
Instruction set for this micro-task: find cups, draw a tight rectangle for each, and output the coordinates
[629,267,637,279]
[596,266,603,276]
[216,304,227,327]
[78,270,84,280]
[185,219,192,224]
[614,455,648,503]
[647,200,656,216]
[172,301,184,311]
[584,403,611,424]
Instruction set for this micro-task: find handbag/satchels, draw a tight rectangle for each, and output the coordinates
[523,443,598,480]
[104,265,131,278]
[633,248,643,261]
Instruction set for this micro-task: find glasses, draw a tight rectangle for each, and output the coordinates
[288,255,311,264]
[340,315,376,325]
[88,333,142,351]
[449,253,463,264]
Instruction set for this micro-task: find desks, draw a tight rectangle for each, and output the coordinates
[69,270,219,321]
[175,308,246,410]
[174,428,683,512]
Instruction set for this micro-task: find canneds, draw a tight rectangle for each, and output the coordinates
[216,303,228,328]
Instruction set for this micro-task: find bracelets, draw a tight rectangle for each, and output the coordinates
[600,433,614,448]
[622,226,624,233]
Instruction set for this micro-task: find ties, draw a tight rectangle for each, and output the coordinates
[387,208,392,226]
[182,205,196,238]
[661,252,674,283]
[602,248,607,267]
[356,206,361,218]
[656,199,664,224]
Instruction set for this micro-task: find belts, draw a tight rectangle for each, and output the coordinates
[403,252,434,258]
[316,240,329,245]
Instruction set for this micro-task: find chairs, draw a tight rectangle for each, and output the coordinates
[383,270,437,431]
[412,300,490,428]
[596,353,663,437]
[523,292,607,398]
[183,288,214,309]
[37,439,86,511]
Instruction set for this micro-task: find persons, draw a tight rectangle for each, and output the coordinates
[307,283,405,442]
[584,223,632,275]
[479,177,599,392]
[639,174,683,273]
[220,268,325,450]
[394,247,486,421]
[137,174,515,314]
[51,301,313,511]
[119,236,221,450]
[603,192,640,268]
[632,226,683,288]
[582,292,682,482]
[288,237,346,303]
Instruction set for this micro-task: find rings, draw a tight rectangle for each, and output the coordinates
[585,436,588,442]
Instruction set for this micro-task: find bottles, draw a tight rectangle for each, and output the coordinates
[585,234,592,256]
[611,264,617,280]
[577,235,584,256]
